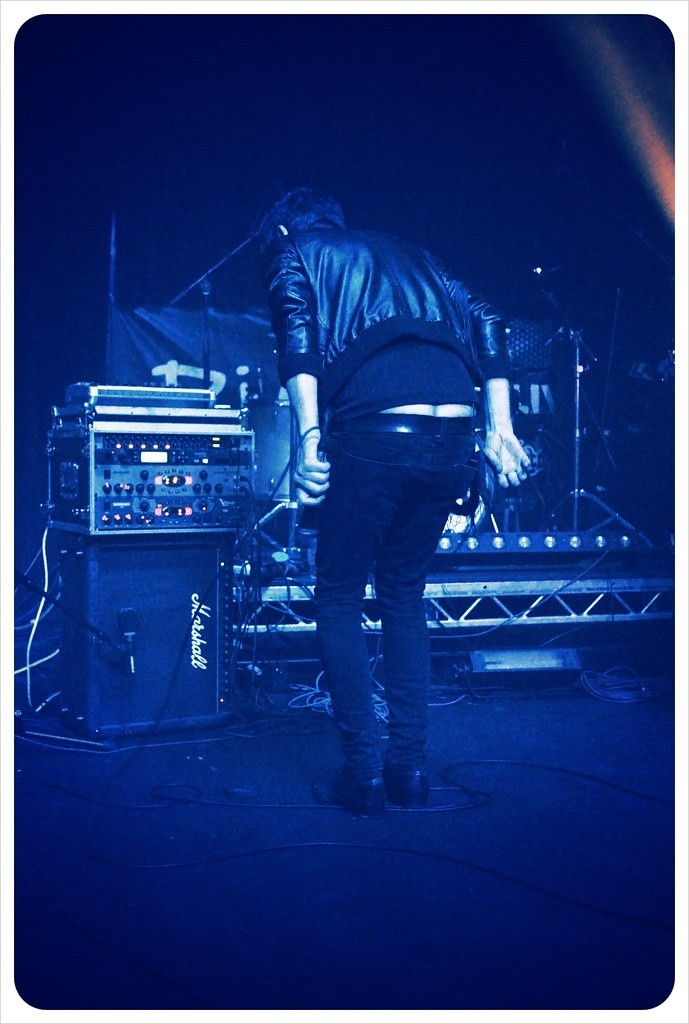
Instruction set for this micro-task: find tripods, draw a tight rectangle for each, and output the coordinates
[537,349,651,548]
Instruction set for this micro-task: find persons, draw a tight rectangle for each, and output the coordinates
[250,185,531,819]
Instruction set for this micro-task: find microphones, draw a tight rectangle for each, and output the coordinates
[118,604,143,679]
[541,324,565,346]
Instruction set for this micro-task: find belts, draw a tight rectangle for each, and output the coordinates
[328,416,482,438]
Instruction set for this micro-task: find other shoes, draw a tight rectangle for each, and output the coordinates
[381,768,431,805]
[311,773,384,814]
